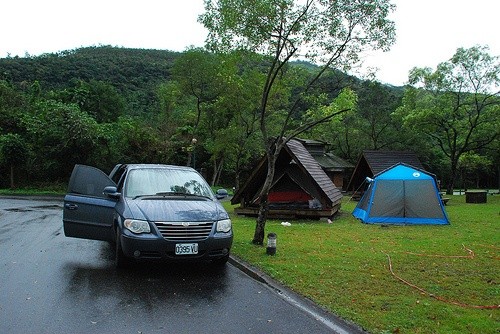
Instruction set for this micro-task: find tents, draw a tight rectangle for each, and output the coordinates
[351,161,451,227]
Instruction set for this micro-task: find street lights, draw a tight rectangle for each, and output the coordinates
[192,138,197,169]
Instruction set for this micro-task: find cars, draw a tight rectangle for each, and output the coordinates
[63,162,234,267]
